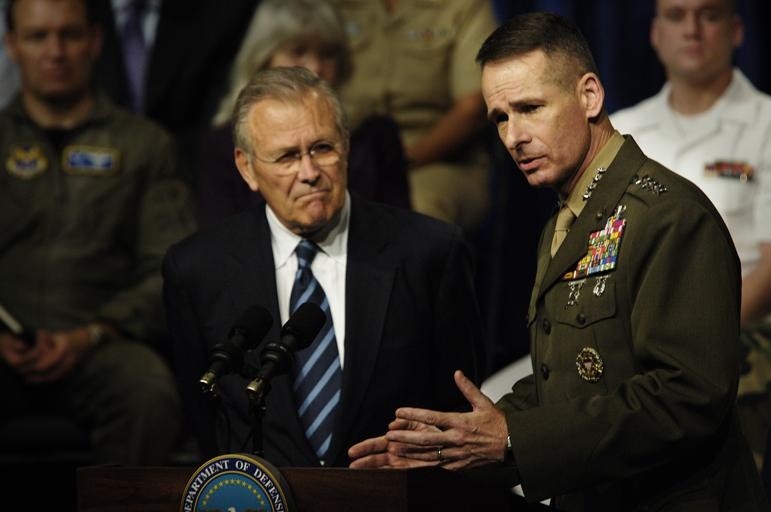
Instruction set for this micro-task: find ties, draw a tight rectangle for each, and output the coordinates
[289,240,342,462]
[550,205,574,257]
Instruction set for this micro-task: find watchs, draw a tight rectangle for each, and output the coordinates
[508,432,511,447]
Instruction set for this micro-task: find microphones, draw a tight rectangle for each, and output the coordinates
[199,304,273,394]
[244,303,326,400]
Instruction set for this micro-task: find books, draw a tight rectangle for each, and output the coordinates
[0,306,34,347]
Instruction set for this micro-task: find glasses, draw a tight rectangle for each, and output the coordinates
[250,146,340,176]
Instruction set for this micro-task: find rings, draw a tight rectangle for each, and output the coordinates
[438,449,440,460]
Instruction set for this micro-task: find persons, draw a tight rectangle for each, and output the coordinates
[177,1,410,235]
[348,13,770,511]
[85,0,209,131]
[0,3,180,469]
[161,67,508,509]
[604,0,770,492]
[213,0,502,230]
